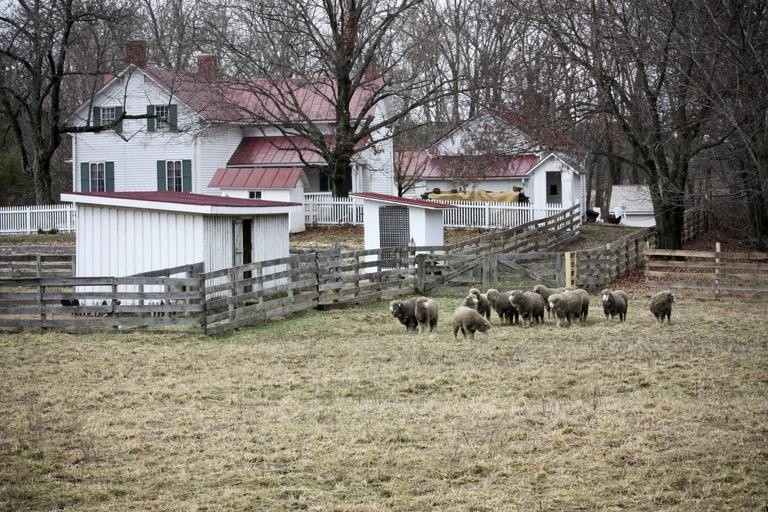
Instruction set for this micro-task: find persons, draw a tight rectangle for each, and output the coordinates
[518,187,532,205]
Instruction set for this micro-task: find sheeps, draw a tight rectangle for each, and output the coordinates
[451,283,591,341]
[599,289,629,323]
[389,296,438,334]
[648,290,676,324]
